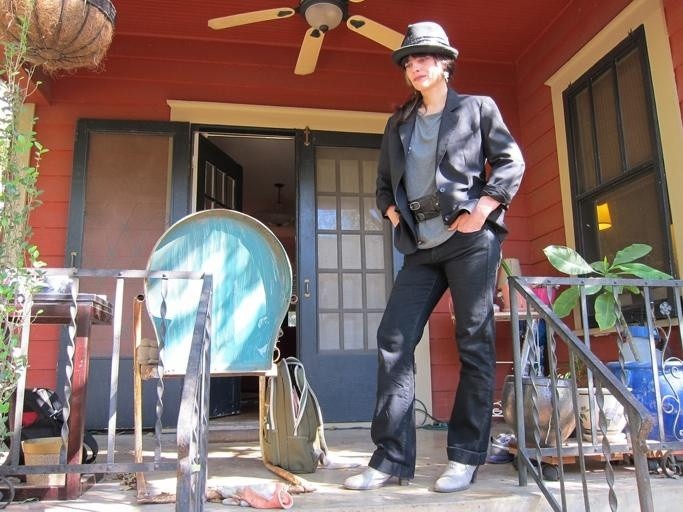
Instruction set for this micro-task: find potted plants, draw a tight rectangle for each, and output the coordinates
[542,243,683,443]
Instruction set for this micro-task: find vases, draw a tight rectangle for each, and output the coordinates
[577,387,633,442]
[502,374,580,448]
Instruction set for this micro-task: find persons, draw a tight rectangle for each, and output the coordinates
[344,20,526,492]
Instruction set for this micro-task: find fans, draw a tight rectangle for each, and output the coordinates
[207,0,407,76]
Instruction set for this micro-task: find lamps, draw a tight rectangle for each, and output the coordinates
[255,183,296,226]
[305,3,343,33]
[596,202,613,231]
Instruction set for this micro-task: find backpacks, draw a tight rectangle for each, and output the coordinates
[263,354,323,475]
[4,380,98,477]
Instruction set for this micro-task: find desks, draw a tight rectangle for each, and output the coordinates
[451,312,543,377]
[0,292,115,501]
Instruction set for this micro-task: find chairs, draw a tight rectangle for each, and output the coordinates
[132,207,318,505]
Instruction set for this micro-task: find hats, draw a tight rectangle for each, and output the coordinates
[391,20,459,63]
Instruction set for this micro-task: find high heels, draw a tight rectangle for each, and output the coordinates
[344,464,409,492]
[434,456,479,493]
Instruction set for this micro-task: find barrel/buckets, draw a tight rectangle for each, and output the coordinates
[499,285,527,313]
[20,436,66,486]
[604,359,683,441]
[532,287,557,311]
[497,257,521,290]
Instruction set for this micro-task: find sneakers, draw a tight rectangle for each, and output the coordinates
[486,431,518,465]
